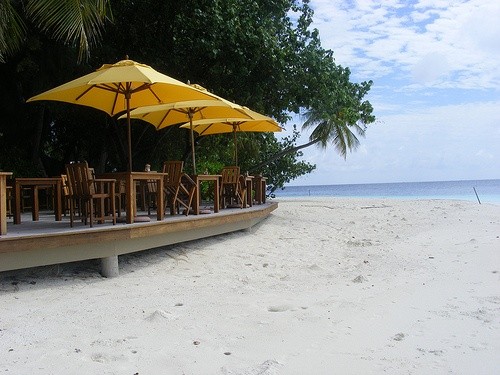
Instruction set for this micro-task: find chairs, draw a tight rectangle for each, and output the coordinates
[6,176,12,220]
[61,160,268,228]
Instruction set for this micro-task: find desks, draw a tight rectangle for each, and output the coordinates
[99,172,168,224]
[0,172,13,235]
[220,176,254,207]
[13,178,63,224]
[253,177,268,205]
[187,174,222,215]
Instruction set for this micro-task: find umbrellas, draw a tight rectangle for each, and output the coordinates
[26,55,286,175]
